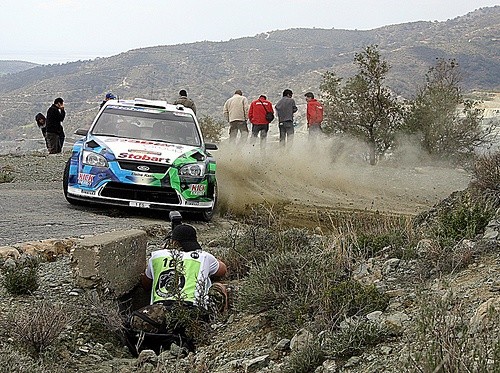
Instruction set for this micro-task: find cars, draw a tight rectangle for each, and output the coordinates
[62,98,219,220]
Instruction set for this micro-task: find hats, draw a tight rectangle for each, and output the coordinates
[106,93,116,99]
[171,222,202,252]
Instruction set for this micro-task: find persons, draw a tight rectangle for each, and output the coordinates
[47,98,66,154]
[131,225,226,335]
[99,94,116,111]
[222,90,323,153]
[35,113,47,140]
[172,90,196,116]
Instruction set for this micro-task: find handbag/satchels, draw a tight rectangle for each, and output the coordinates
[266,111,274,121]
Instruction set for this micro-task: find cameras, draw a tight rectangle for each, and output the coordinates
[169,211,182,231]
